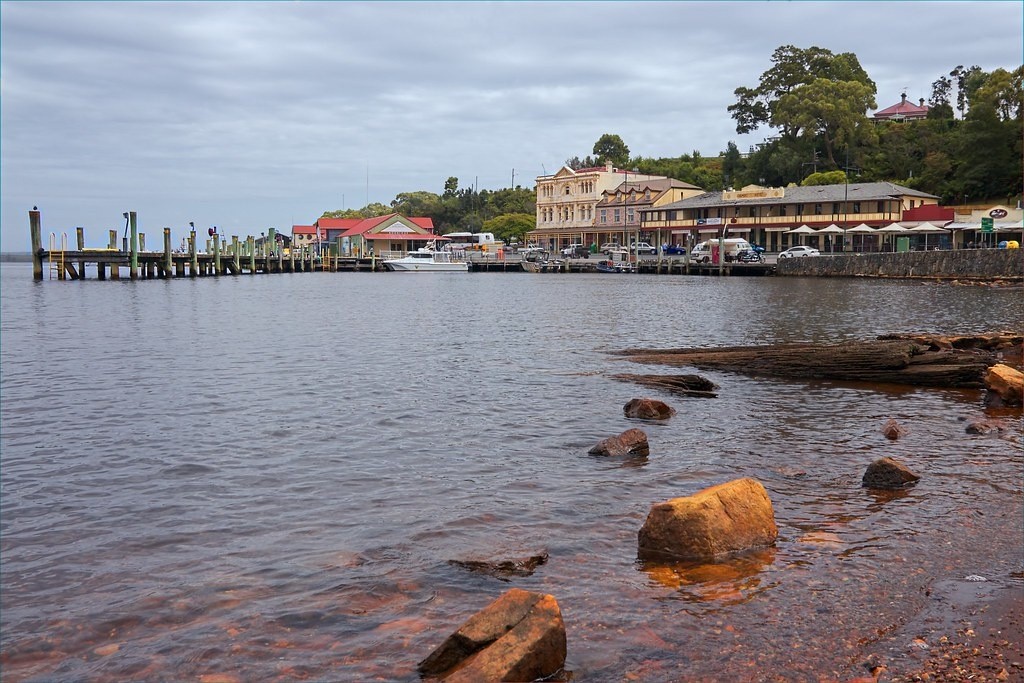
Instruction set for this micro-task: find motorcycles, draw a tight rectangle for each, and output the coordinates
[737,244,767,265]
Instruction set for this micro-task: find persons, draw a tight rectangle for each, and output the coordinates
[662,241,667,255]
[370,245,373,256]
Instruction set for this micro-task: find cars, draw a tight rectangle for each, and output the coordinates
[654,244,686,256]
[270,248,314,258]
[777,245,821,259]
[501,241,550,263]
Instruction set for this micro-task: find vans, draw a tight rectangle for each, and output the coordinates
[690,239,753,264]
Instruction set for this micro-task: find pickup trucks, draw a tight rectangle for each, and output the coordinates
[560,243,592,260]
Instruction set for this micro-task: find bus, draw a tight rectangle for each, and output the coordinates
[436,231,495,253]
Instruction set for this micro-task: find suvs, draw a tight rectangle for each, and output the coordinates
[599,243,628,256]
[630,241,656,255]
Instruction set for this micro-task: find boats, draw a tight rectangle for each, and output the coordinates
[595,259,640,274]
[382,230,473,273]
[520,245,572,274]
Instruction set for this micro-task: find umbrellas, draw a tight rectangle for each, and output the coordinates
[784,221,1024,252]
